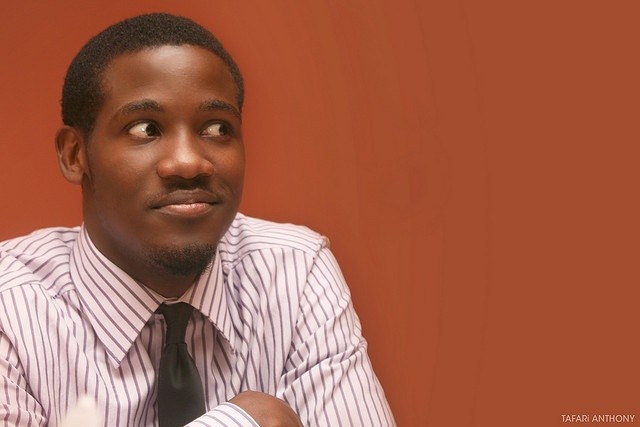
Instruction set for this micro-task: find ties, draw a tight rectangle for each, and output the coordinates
[153,302,204,427]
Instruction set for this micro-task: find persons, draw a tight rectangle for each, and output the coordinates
[1,13,399,427]
